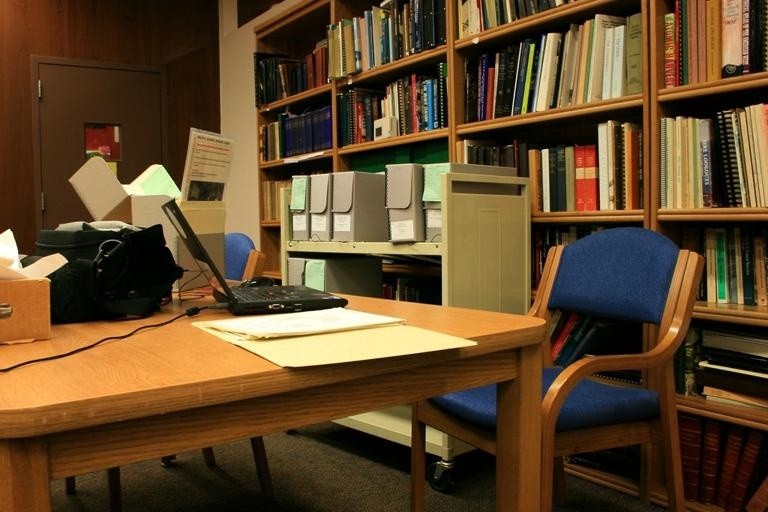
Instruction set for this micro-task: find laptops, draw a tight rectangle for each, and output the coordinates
[161,198,349,316]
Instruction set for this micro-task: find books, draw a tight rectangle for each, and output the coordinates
[673,322,768,410]
[261,178,292,222]
[664,1,768,88]
[464,13,644,124]
[456,120,643,214]
[455,1,577,40]
[660,103,768,208]
[661,414,768,511]
[179,125,235,203]
[673,224,768,305]
[253,0,450,163]
[531,223,642,481]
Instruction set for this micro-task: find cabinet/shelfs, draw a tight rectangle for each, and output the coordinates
[253,0,767,512]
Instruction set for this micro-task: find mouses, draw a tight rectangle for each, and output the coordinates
[248,277,274,286]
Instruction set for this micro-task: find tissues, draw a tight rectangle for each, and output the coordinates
[0,245,52,343]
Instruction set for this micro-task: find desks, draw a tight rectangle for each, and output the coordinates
[0,283,549,512]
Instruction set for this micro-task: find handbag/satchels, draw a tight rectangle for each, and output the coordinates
[35,224,183,324]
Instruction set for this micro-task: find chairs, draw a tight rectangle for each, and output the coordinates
[65,230,271,512]
